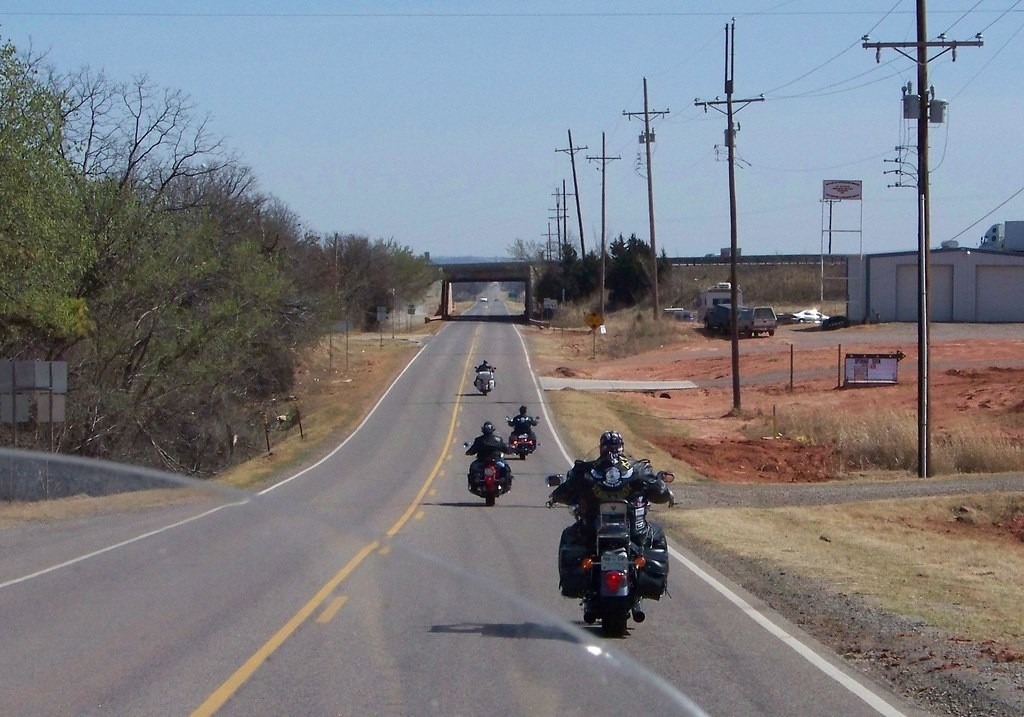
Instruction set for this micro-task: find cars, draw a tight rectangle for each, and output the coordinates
[790,309,830,324]
[662,310,694,323]
[480,296,488,302]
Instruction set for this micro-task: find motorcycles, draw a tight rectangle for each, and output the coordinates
[474,366,496,397]
[544,445,675,637]
[505,415,540,461]
[462,435,515,507]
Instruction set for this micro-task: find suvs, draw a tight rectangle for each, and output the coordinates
[730,306,777,339]
[703,303,748,338]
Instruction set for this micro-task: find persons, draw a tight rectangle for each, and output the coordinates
[508,405,537,449]
[474,359,496,387]
[466,421,510,491]
[552,431,675,598]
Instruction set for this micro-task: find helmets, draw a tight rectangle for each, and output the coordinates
[600,430,624,447]
[481,421,495,430]
[519,406,526,411]
